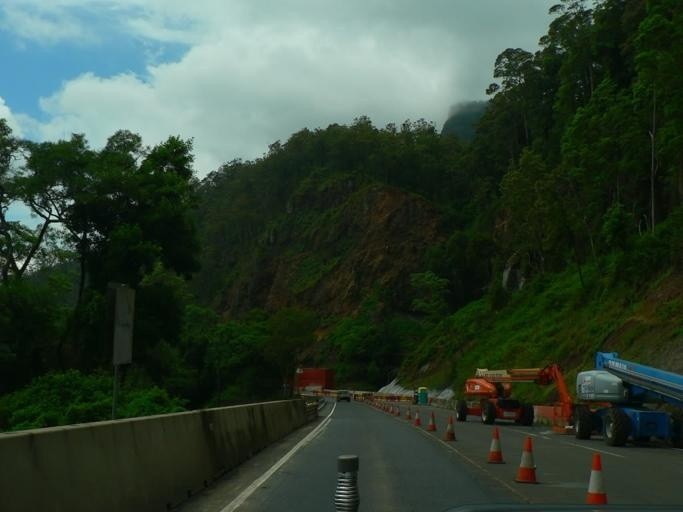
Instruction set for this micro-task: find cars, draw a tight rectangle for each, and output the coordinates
[336,389,353,402]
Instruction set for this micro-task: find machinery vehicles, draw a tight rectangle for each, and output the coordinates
[456,360,577,425]
[576,351,683,448]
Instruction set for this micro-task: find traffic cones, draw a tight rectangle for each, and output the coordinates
[440,415,457,442]
[364,398,437,431]
[487,426,506,464]
[584,451,609,512]
[514,434,539,484]
